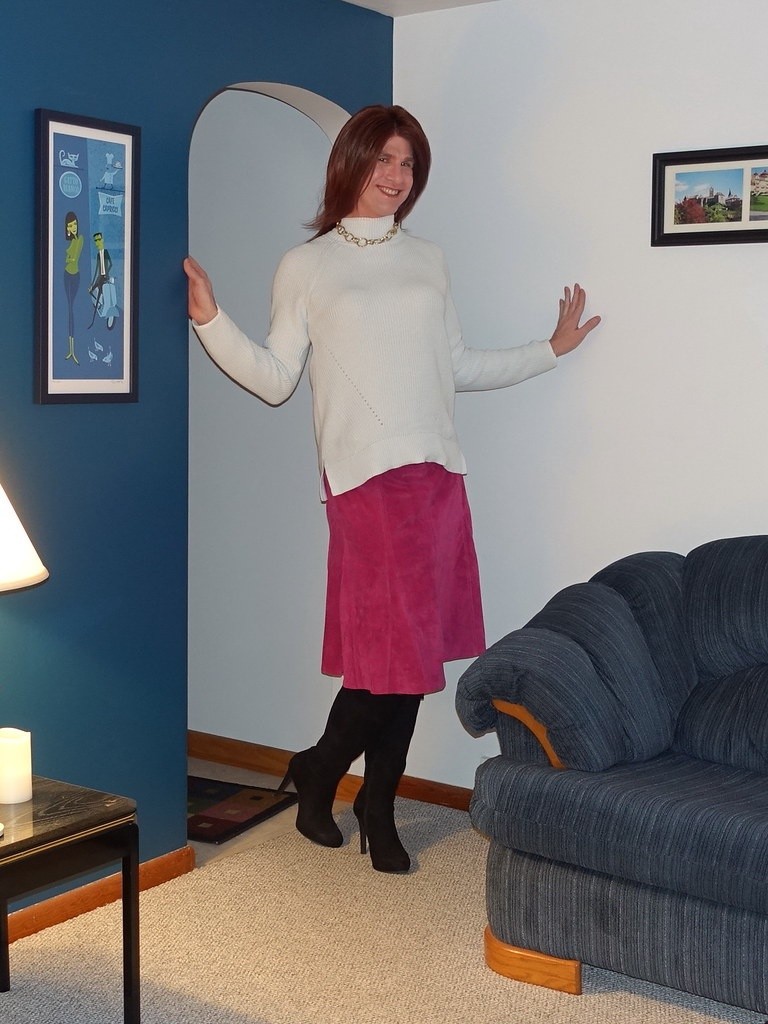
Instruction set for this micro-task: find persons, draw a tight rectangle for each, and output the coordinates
[184,105,601,873]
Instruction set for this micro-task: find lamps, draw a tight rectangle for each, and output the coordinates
[0,483,49,593]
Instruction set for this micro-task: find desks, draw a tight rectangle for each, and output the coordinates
[0,774,140,1024]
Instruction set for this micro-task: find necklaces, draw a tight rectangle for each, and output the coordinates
[336,221,399,247]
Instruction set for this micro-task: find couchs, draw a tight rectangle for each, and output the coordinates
[453,534,768,1014]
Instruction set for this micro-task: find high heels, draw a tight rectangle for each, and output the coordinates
[353,785,411,874]
[275,746,344,849]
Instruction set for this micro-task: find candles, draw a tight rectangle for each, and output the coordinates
[0,727,33,805]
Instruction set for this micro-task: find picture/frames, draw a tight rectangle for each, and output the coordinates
[651,145,768,247]
[33,108,140,406]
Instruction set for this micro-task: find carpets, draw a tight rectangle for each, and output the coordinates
[187,775,298,845]
[0,794,768,1024]
